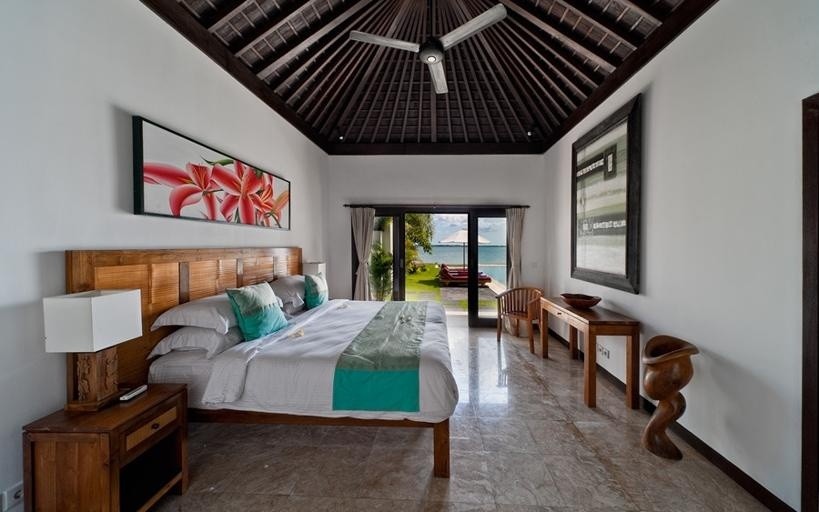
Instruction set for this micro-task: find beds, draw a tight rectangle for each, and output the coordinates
[65,244,460,478]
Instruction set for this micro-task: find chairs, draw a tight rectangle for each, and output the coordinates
[437,262,492,286]
[495,286,549,353]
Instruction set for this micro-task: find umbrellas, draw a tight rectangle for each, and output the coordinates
[438,227,491,271]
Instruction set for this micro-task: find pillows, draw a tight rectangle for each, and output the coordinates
[282,302,302,314]
[304,272,329,309]
[149,292,240,336]
[145,326,245,359]
[225,281,288,342]
[269,275,307,307]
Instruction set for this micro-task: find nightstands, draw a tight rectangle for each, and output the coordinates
[22,380,189,510]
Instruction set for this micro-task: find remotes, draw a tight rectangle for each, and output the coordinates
[120,384,148,401]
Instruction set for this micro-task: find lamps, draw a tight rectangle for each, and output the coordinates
[43,288,144,414]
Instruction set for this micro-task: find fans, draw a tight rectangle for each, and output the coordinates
[350,1,508,97]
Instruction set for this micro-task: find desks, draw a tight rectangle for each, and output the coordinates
[538,295,643,409]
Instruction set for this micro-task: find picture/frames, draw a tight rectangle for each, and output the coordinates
[132,114,290,231]
[570,92,643,294]
